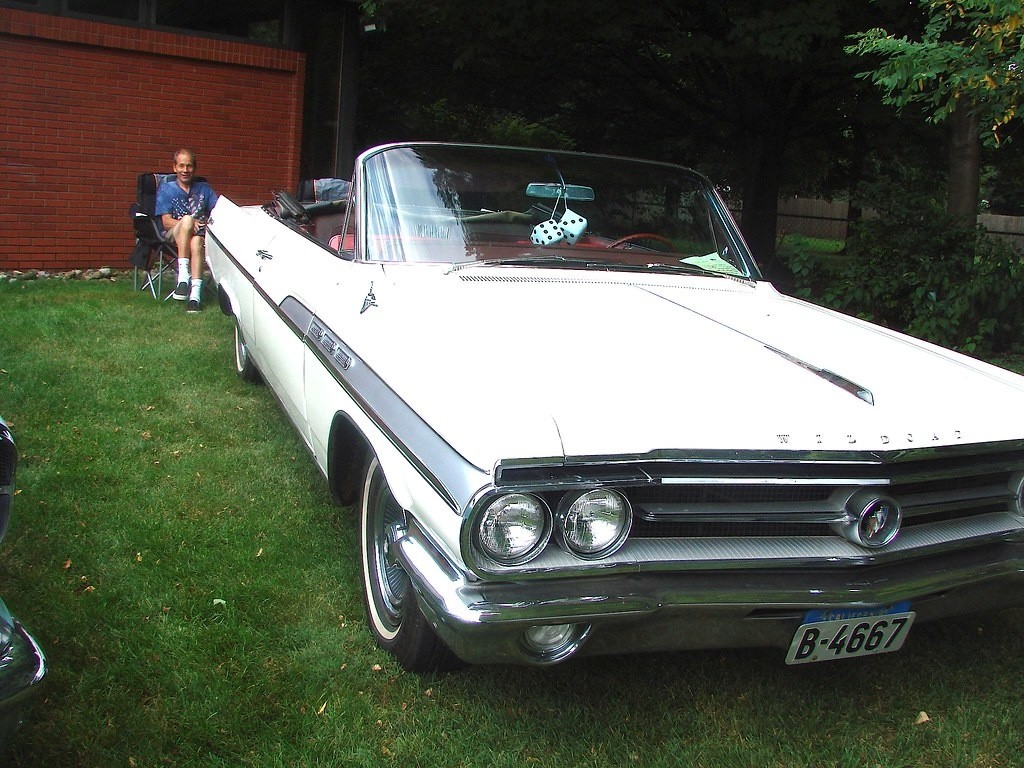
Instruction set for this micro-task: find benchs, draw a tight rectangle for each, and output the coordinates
[314,215,700,266]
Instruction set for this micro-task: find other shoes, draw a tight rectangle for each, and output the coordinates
[172,282,189,300]
[187,300,201,313]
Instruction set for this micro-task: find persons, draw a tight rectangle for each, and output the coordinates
[155,149,218,313]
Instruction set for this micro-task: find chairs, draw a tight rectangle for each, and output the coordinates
[129,173,218,302]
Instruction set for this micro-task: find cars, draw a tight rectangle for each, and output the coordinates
[200,140,1024,673]
